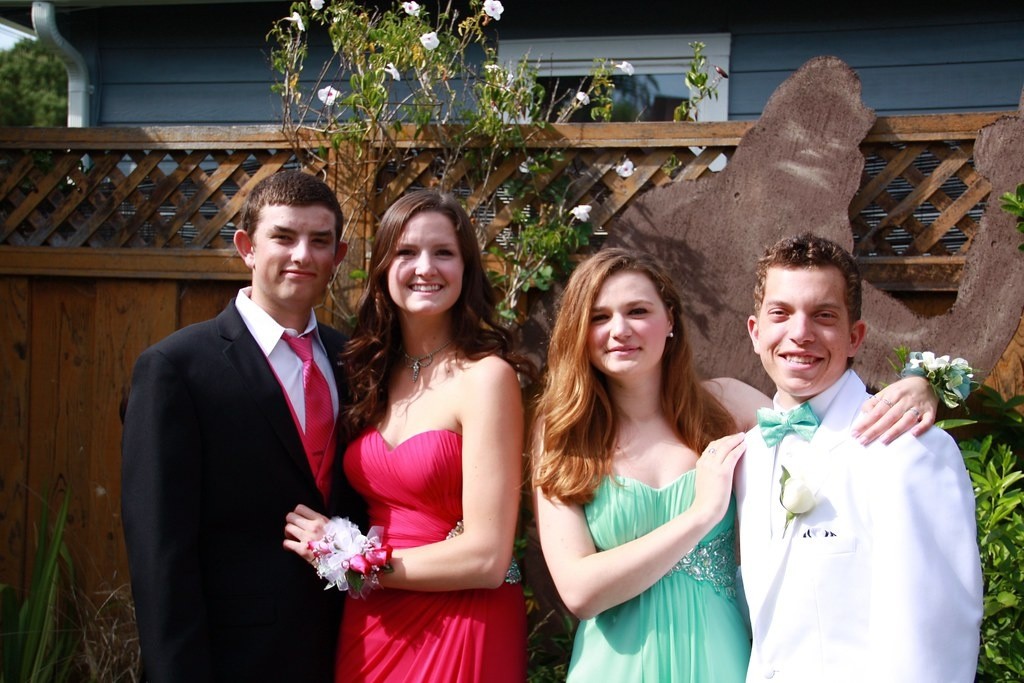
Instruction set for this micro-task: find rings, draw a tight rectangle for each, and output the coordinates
[884,399,893,407]
[709,449,716,454]
[872,396,877,398]
[911,408,922,417]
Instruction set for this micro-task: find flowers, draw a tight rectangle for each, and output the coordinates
[260,0,638,323]
[778,465,816,538]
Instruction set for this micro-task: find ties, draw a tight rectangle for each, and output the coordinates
[283,330,333,468]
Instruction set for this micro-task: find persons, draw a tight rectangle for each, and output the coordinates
[530,247,984,683]
[285,190,524,683]
[120,171,369,682]
[736,232,984,683]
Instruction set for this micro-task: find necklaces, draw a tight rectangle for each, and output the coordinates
[403,340,452,383]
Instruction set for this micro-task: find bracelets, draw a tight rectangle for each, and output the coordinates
[307,516,393,601]
[886,347,981,408]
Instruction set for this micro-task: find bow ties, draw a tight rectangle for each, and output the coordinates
[756,402,819,448]
